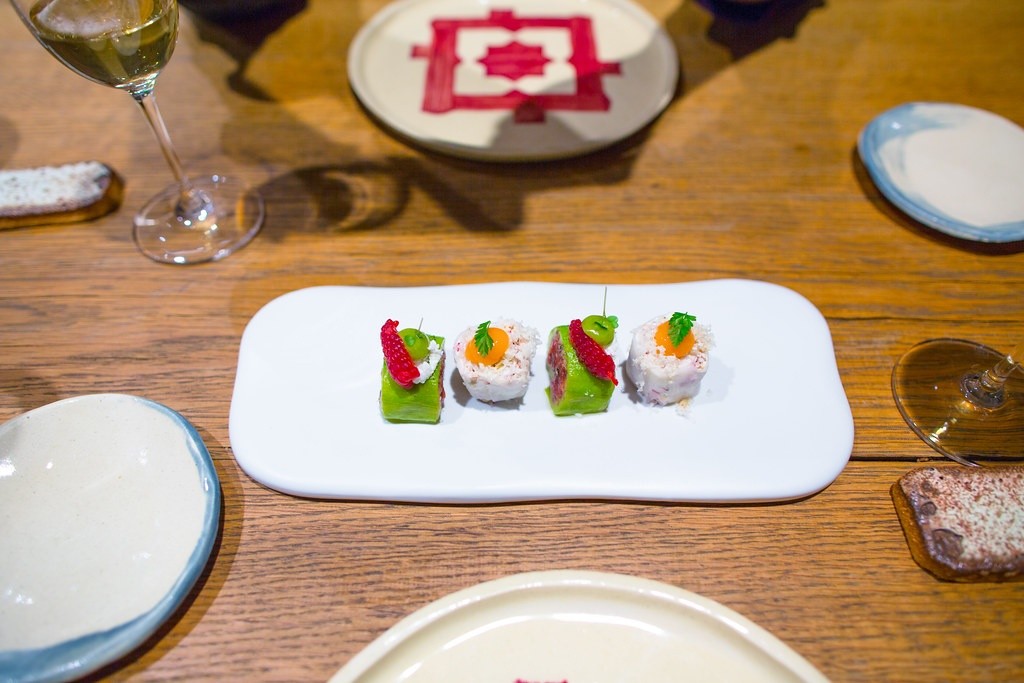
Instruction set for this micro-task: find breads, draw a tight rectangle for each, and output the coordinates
[890,462,1024,582]
[0,158,127,230]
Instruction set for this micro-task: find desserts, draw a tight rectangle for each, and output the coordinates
[377,284,715,422]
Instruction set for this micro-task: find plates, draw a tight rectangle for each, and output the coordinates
[227,278,855,505]
[857,101,1024,244]
[327,569,831,683]
[344,0,680,163]
[0,393,222,683]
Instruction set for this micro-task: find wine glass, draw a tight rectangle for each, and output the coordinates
[6,0,264,266]
[892,337,1024,470]
